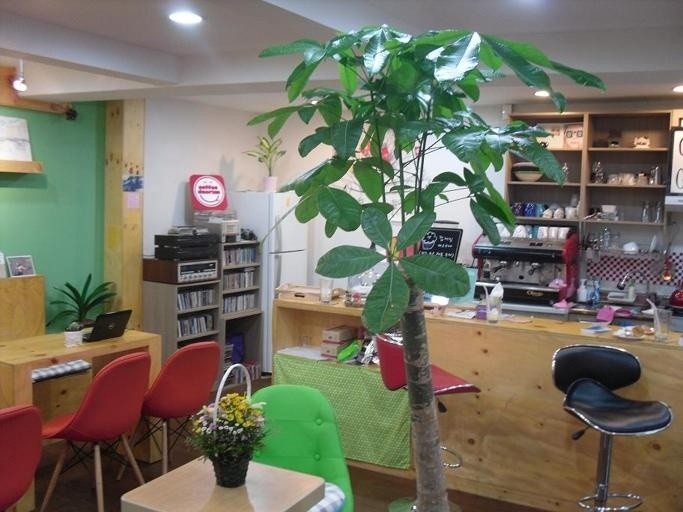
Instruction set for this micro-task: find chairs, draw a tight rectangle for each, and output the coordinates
[114,339,222,483]
[243,382,354,511]
[374,332,481,510]
[548,340,674,512]
[0,400,42,510]
[37,351,152,511]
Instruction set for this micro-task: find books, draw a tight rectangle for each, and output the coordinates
[177,247,267,386]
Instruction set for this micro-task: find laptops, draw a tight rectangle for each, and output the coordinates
[82,309,132,342]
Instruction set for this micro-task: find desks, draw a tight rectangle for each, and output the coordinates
[0,325,164,512]
[119,441,325,511]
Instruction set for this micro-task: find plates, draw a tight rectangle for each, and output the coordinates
[615,332,642,340]
[624,326,655,334]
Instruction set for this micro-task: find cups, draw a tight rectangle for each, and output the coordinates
[513,201,545,219]
[592,172,605,184]
[512,224,571,241]
[638,176,648,185]
[475,304,486,320]
[318,278,333,304]
[485,295,501,324]
[543,207,577,220]
[654,308,673,343]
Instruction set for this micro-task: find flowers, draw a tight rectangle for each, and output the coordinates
[184,391,268,464]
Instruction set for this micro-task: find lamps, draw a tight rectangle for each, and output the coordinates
[10,59,28,93]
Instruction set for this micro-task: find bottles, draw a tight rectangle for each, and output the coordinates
[611,234,621,256]
[562,162,569,184]
[654,166,662,185]
[576,279,588,303]
[627,280,634,299]
[602,227,612,250]
[591,280,601,305]
[641,201,662,224]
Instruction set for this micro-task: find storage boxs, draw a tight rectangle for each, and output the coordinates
[320,324,357,343]
[320,341,353,360]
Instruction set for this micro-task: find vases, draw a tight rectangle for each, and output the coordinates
[207,451,253,486]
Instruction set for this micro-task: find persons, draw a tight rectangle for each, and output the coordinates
[14,263,33,275]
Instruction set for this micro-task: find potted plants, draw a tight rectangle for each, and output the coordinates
[240,132,288,192]
[42,273,118,329]
[62,322,83,348]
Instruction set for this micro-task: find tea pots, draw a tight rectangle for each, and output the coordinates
[668,288,683,306]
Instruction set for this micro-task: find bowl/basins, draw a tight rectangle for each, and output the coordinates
[600,204,616,213]
[622,242,639,255]
[512,170,545,181]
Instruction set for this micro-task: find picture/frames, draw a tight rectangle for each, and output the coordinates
[4,254,36,278]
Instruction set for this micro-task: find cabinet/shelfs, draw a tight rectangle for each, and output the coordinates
[216,236,263,390]
[503,108,669,224]
[140,277,220,391]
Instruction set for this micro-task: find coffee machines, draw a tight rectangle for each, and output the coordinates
[471,229,580,306]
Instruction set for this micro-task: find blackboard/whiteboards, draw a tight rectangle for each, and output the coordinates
[417,228,463,263]
